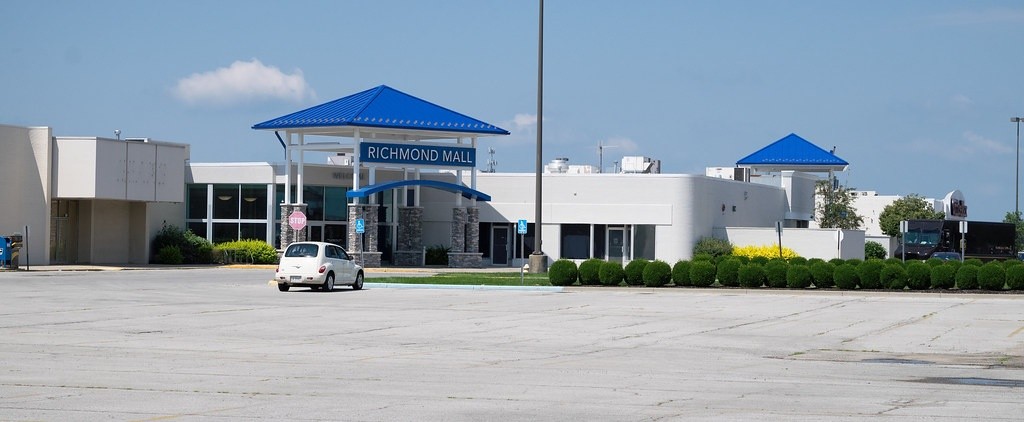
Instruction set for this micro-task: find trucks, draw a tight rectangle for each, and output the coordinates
[893,219,1017,264]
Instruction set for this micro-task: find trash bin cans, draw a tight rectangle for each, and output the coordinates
[0,236,12,268]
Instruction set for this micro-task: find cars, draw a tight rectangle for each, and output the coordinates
[1018,252,1024,262]
[274,241,365,292]
[930,252,962,263]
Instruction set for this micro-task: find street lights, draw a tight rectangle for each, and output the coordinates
[1009,117,1024,219]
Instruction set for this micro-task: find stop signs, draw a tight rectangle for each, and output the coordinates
[288,211,307,231]
